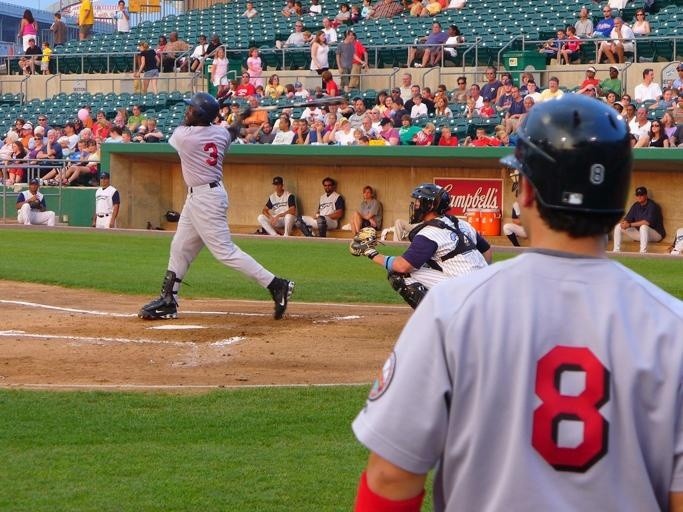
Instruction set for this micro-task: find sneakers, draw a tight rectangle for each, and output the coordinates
[137,298,178,320]
[35,178,71,186]
[273,279,296,320]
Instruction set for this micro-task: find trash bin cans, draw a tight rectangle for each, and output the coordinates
[502,50,548,89]
[166,211,179,231]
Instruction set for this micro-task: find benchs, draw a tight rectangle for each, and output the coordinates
[162,219,676,255]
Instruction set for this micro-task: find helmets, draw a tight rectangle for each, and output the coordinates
[410,183,450,216]
[184,91,219,122]
[499,93,632,218]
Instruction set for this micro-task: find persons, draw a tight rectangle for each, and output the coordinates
[293,177,345,238]
[255,174,298,238]
[501,199,528,247]
[134,91,296,322]
[391,218,421,240]
[15,178,55,227]
[609,186,666,254]
[541,1,662,66]
[349,94,683,512]
[91,171,120,229]
[242,1,467,88]
[211,62,682,150]
[348,186,383,236]
[350,183,492,313]
[132,32,230,89]
[17,1,131,75]
[0,105,163,186]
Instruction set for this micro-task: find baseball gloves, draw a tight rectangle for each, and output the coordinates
[348,227,377,256]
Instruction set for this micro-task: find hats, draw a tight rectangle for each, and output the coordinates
[634,186,647,196]
[378,117,390,126]
[272,177,283,185]
[294,81,302,88]
[586,67,595,73]
[676,63,683,70]
[339,117,347,128]
[100,172,109,178]
[29,179,38,185]
[614,101,624,110]
[22,124,32,130]
[610,65,620,74]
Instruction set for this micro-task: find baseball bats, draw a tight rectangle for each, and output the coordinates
[250,96,343,112]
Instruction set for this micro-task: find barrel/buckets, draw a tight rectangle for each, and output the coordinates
[479,207,502,237]
[466,207,479,232]
